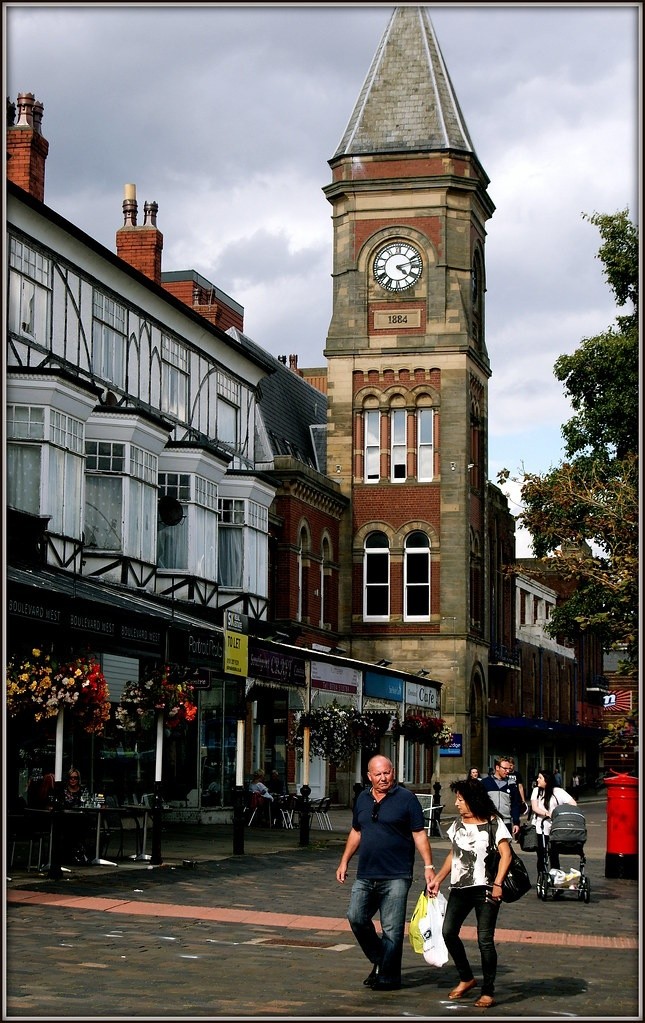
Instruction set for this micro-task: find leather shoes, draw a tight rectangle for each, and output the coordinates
[363,962,380,985]
[371,982,402,992]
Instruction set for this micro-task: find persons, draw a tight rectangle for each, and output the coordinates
[530,771,578,875]
[30,769,91,866]
[335,755,436,991]
[468,756,528,840]
[532,769,580,801]
[427,778,513,1007]
[249,770,285,828]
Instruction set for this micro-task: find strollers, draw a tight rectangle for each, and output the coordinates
[536,804,590,905]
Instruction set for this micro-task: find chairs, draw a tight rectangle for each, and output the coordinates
[244,790,334,830]
[8,790,157,872]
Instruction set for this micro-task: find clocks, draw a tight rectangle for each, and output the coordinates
[373,241,423,293]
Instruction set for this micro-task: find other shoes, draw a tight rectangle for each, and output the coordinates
[448,979,476,1000]
[474,995,493,1008]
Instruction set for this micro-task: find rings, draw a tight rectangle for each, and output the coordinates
[498,898,501,901]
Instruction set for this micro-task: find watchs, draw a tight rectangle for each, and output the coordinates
[424,864,435,870]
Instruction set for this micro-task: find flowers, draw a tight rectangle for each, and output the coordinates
[114,661,208,738]
[391,712,455,751]
[283,697,380,767]
[6,639,112,738]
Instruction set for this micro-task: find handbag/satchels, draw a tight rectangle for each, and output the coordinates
[553,868,581,889]
[519,824,538,852]
[419,890,450,967]
[408,891,425,955]
[482,819,533,904]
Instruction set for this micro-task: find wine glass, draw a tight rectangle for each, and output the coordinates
[80,792,86,807]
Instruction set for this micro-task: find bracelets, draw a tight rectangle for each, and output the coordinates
[493,883,502,887]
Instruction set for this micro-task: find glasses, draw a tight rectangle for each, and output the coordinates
[68,775,78,781]
[372,802,380,822]
[498,765,512,772]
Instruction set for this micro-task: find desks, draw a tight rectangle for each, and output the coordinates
[72,806,128,868]
[123,805,171,861]
[23,808,84,874]
